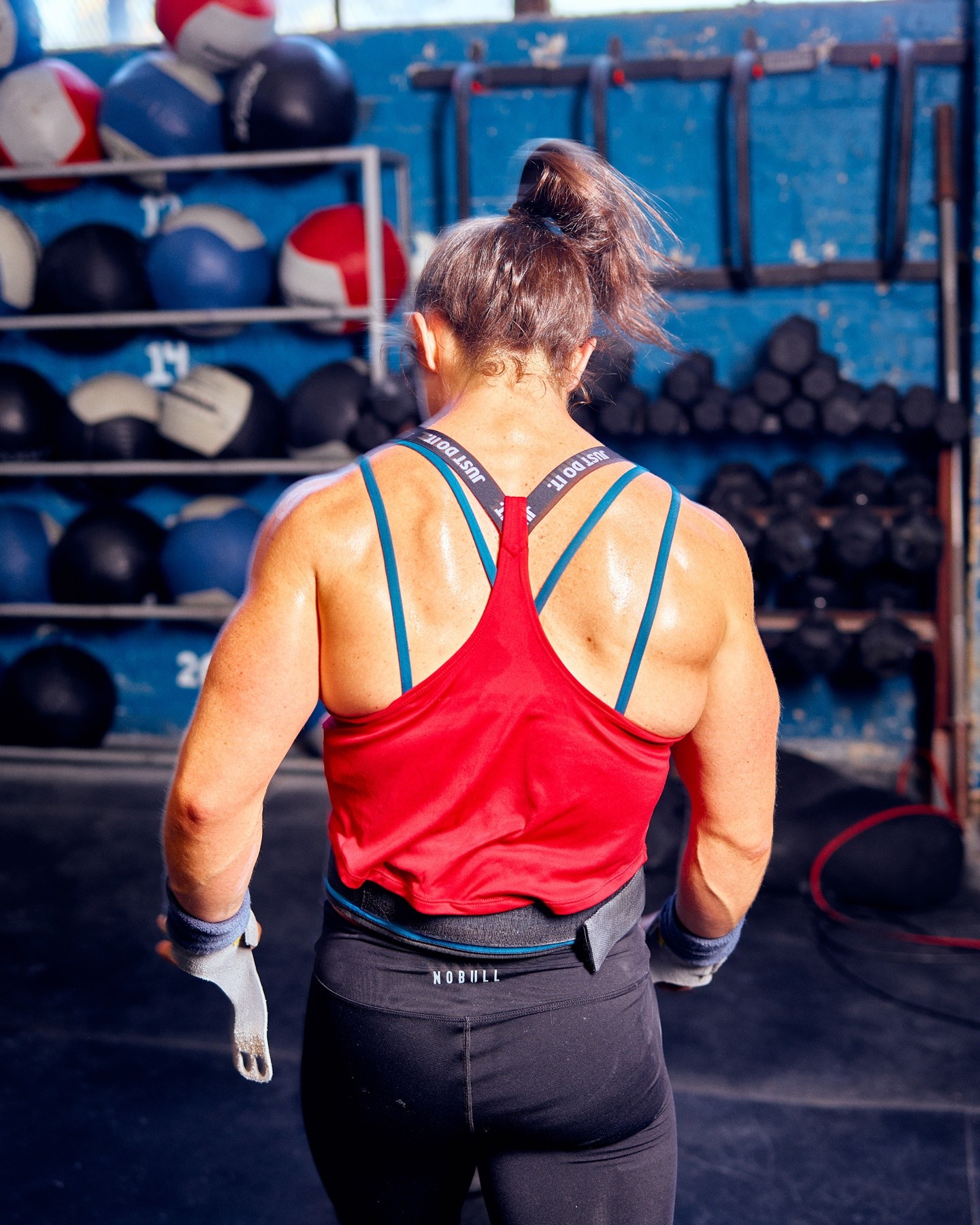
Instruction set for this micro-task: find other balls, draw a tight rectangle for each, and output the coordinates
[0,0,413,750]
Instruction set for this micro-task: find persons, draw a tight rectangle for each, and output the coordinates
[163,137,788,1225]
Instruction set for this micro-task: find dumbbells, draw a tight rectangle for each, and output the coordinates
[566,313,971,690]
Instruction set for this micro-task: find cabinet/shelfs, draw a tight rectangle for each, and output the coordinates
[754,450,952,723]
[0,144,410,790]
